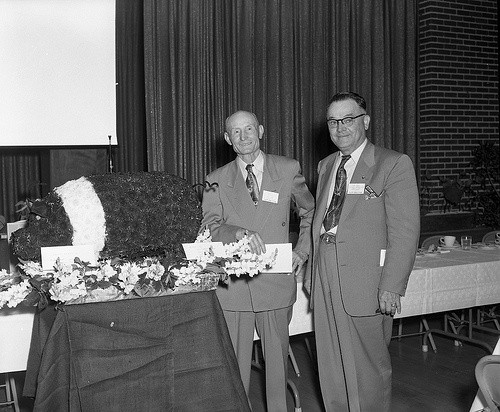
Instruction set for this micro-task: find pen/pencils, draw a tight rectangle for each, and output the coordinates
[379,189,385,198]
[366,184,378,197]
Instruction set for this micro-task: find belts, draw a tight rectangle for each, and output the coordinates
[319,234,337,244]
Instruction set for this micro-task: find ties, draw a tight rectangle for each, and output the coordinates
[245,164,260,207]
[322,155,351,232]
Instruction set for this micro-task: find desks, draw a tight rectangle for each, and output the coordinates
[0,243,500,412]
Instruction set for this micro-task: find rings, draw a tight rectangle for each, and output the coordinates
[391,303,395,307]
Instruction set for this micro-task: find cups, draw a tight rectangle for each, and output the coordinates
[439,235,456,246]
[460,236,473,250]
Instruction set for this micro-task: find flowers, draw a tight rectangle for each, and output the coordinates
[0,224,278,311]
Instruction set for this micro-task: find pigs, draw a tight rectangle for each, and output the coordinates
[11,173,218,268]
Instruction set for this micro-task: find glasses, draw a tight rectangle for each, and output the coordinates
[325,114,366,129]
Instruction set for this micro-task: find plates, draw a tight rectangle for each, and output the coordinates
[416,242,500,258]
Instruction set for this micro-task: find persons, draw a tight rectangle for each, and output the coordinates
[197,110,315,411]
[303,92,420,412]
